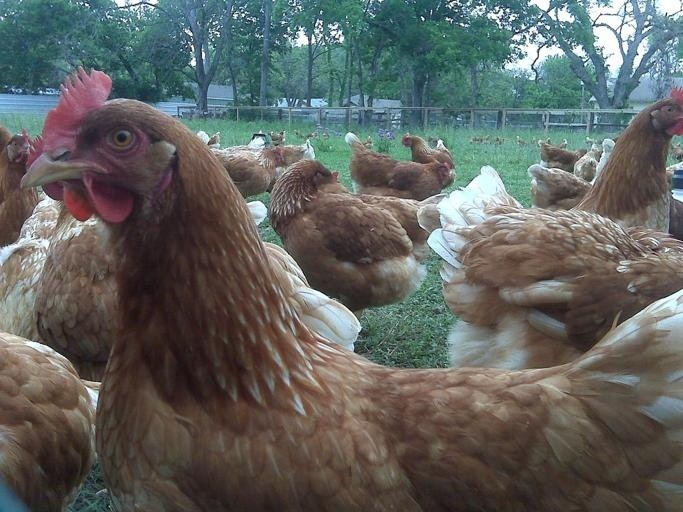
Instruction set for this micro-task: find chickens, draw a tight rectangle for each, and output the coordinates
[0,65,683,512]
